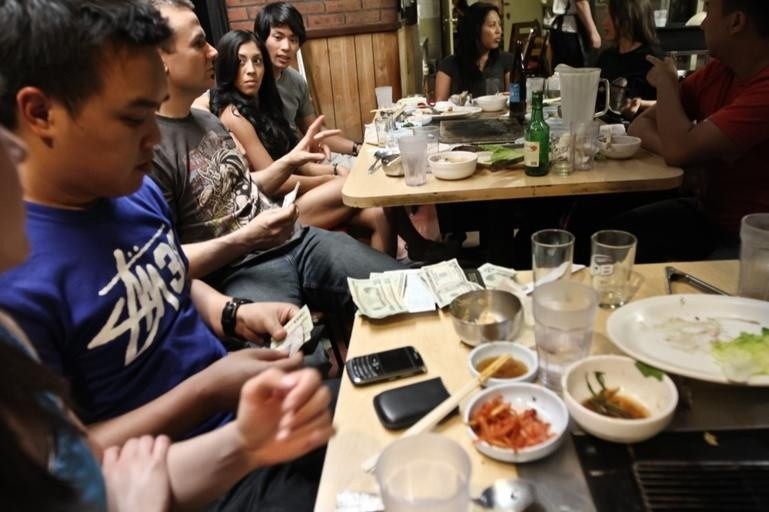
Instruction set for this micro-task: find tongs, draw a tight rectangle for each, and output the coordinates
[663,265,733,410]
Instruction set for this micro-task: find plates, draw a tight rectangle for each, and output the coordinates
[607,294,769,390]
[401,98,482,125]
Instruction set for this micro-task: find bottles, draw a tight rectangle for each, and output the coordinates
[523,90,550,177]
[507,40,526,119]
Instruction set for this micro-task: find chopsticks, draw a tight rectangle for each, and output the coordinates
[603,133,612,149]
[362,350,511,471]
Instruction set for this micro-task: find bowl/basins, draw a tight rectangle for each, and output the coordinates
[451,290,523,346]
[476,95,508,112]
[380,155,405,178]
[467,383,569,462]
[427,151,478,180]
[474,105,565,172]
[599,137,642,158]
[563,354,678,448]
[469,337,536,390]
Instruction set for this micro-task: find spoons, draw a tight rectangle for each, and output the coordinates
[337,478,538,511]
[369,150,387,173]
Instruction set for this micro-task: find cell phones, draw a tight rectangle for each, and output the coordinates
[346,347,426,385]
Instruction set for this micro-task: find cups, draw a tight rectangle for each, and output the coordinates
[737,213,769,300]
[374,116,396,148]
[534,280,601,389]
[551,124,575,176]
[572,121,597,172]
[397,134,429,187]
[379,436,473,511]
[414,128,439,172]
[590,229,638,309]
[374,85,394,111]
[530,229,575,285]
[527,78,560,102]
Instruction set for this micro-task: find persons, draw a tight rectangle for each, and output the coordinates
[591,0,667,120]
[622,0,769,262]
[430,0,519,102]
[537,0,603,73]
[1,127,339,511]
[209,29,400,258]
[250,1,364,156]
[0,1,344,511]
[145,1,421,349]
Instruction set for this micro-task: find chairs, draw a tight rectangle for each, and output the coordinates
[508,20,553,89]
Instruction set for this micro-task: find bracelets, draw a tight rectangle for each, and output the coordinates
[353,139,362,156]
[219,296,253,337]
[330,162,338,176]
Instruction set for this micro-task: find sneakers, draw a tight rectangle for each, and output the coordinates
[406,237,448,262]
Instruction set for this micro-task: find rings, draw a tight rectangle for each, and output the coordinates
[317,143,324,153]
